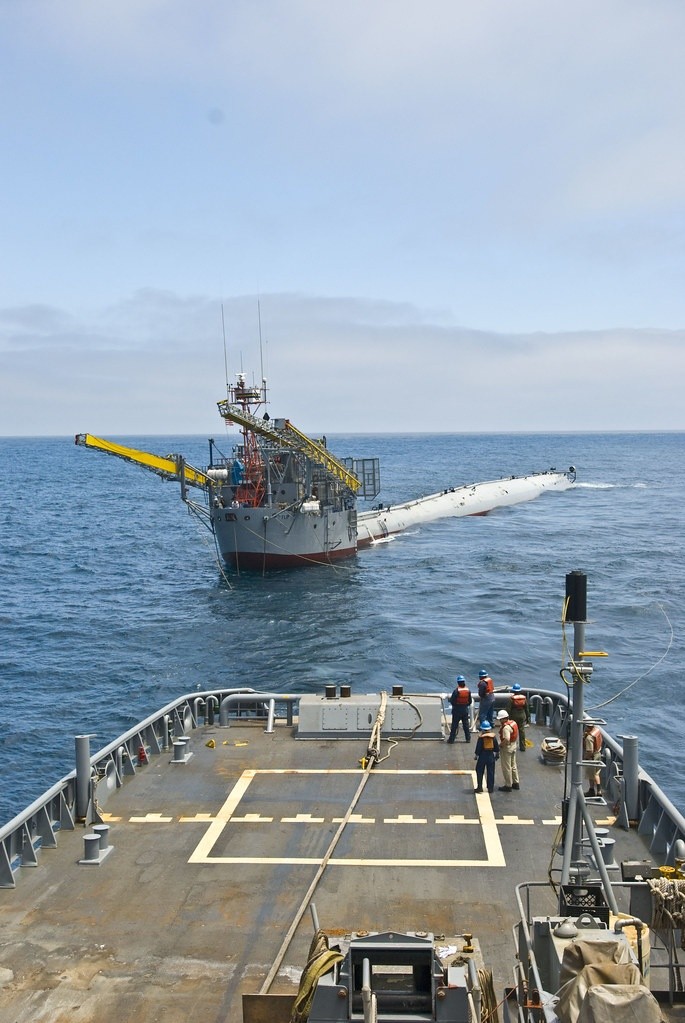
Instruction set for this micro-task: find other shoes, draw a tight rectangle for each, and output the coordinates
[465,739,470,743]
[595,792,602,802]
[498,785,513,792]
[446,739,454,744]
[584,790,595,797]
[511,783,520,790]
[473,787,483,793]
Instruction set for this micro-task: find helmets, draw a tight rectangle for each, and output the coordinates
[585,715,595,727]
[456,674,465,681]
[496,710,509,719]
[478,669,489,676]
[512,683,521,691]
[479,721,492,730]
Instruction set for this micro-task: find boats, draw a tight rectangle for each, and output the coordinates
[72,294,577,580]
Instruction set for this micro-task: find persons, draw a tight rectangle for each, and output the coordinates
[477,670,495,729]
[506,683,530,751]
[447,675,472,743]
[583,723,603,802]
[474,720,500,793]
[496,709,520,793]
